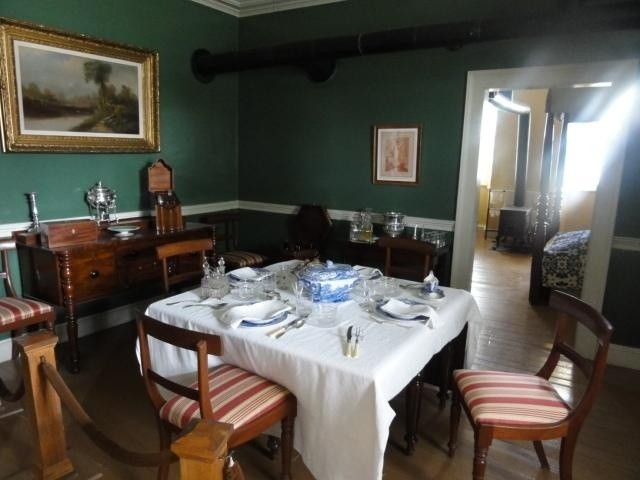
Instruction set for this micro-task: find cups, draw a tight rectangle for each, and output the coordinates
[354,268,401,314]
[316,301,337,327]
[239,262,314,318]
[382,212,405,237]
[350,211,373,242]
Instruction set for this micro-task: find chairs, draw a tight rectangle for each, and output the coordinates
[205,210,268,268]
[155,238,215,297]
[446,285,613,478]
[0,247,56,332]
[126,306,299,478]
[377,235,436,282]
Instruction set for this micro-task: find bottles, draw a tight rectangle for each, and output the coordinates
[424,269,439,293]
[425,228,446,247]
[201,257,229,300]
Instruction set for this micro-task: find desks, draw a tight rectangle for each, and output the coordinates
[343,239,448,282]
[14,216,217,373]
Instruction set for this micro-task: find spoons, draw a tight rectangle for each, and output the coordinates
[275,319,305,339]
[164,298,228,309]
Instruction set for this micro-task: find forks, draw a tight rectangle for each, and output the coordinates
[352,328,361,356]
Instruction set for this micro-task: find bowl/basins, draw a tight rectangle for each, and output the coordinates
[301,259,358,303]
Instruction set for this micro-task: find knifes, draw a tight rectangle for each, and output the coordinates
[267,314,306,337]
[343,326,352,356]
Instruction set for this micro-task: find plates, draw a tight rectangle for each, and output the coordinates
[229,267,270,281]
[372,287,445,323]
[241,312,289,327]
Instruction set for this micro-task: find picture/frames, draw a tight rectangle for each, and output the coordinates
[371,123,423,186]
[0,17,163,155]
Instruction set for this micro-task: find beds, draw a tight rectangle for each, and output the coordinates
[528,83,612,302]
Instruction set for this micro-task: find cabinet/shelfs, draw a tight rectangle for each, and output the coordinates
[492,205,533,252]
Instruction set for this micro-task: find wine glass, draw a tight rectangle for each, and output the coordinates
[29,191,44,233]
